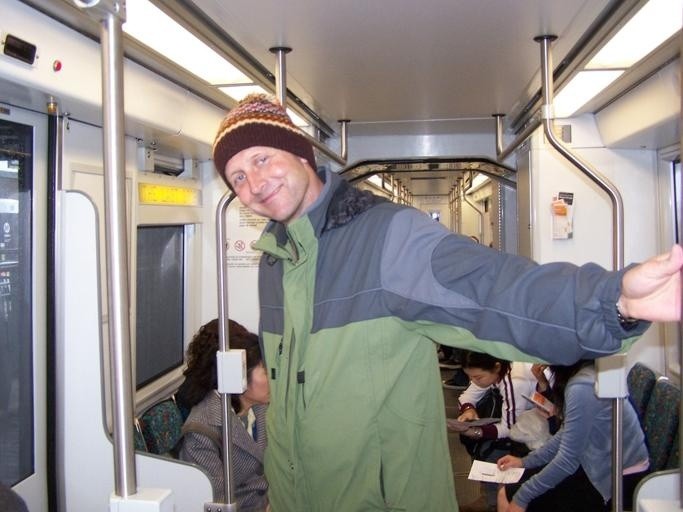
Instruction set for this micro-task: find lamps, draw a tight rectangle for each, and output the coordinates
[583,0,683,71]
[216,84,310,128]
[553,70,629,118]
[120,0,254,86]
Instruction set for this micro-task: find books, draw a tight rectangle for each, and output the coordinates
[521,391,562,419]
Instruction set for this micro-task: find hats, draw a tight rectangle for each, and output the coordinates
[212,91,318,196]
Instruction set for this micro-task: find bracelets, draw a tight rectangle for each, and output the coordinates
[615,301,640,329]
[462,406,473,411]
[471,427,480,442]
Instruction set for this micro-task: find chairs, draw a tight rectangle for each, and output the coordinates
[135,388,185,459]
[641,376,682,472]
[627,362,658,414]
[632,468,683,512]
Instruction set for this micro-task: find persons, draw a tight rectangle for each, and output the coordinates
[211,93,682,511]
[178,333,271,512]
[177,318,250,424]
[458,364,565,511]
[436,343,466,370]
[442,367,470,391]
[454,351,555,464]
[495,358,650,511]
